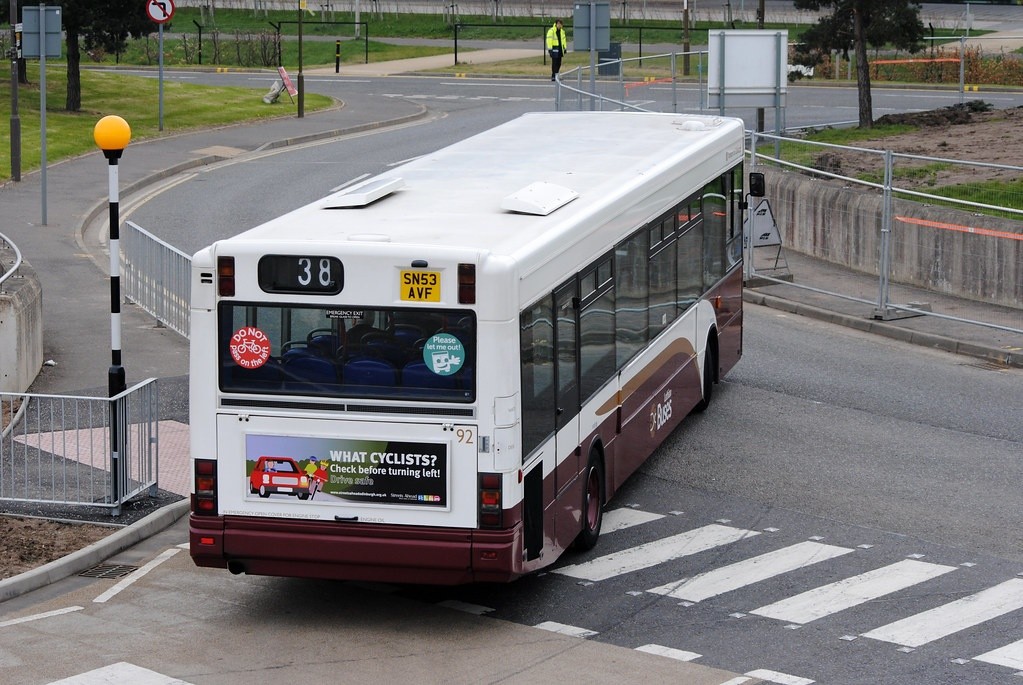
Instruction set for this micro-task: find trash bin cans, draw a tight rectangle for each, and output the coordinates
[598,43,622,76]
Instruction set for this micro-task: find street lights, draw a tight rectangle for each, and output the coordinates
[92,114,133,503]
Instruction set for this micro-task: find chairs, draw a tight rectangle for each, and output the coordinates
[235,311,475,401]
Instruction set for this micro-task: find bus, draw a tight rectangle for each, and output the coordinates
[186,110,765,577]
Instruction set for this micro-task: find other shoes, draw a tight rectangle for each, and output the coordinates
[552,78,555,81]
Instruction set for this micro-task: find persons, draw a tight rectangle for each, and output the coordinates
[344,310,388,344]
[546,20,567,81]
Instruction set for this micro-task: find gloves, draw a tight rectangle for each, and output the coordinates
[549,50,552,56]
[564,50,567,54]
